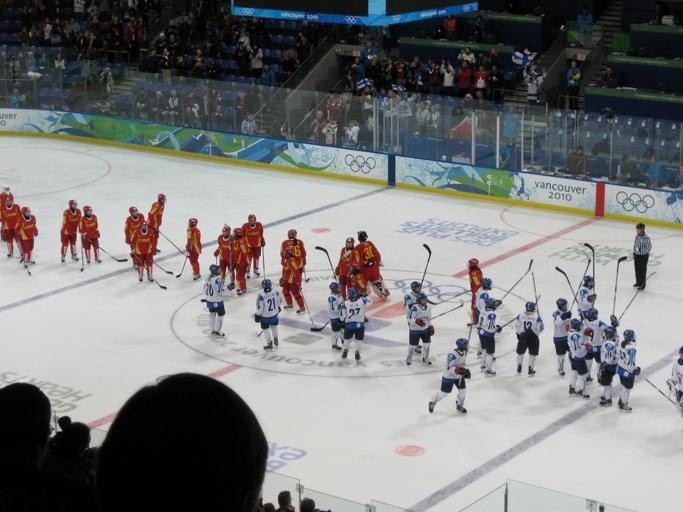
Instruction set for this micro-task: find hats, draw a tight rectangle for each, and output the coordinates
[636,222,645,229]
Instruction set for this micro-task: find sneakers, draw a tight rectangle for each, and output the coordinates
[429,401,435,413]
[192,272,201,280]
[477,350,496,375]
[457,405,467,413]
[227,268,261,295]
[284,304,305,313]
[569,375,593,397]
[7,250,36,267]
[61,254,101,263]
[600,395,631,410]
[633,284,646,290]
[558,368,565,376]
[406,346,432,365]
[332,337,361,360]
[209,329,225,336]
[135,264,153,281]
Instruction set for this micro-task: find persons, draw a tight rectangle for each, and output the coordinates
[0,371,330,511]
[403,282,428,355]
[337,285,374,361]
[60,200,102,263]
[336,231,391,304]
[204,264,227,338]
[666,345,683,412]
[253,278,283,349]
[429,338,473,414]
[405,291,433,367]
[327,279,348,352]
[2,2,682,193]
[474,273,642,411]
[632,222,651,291]
[1,195,38,269]
[214,215,266,295]
[280,230,306,314]
[467,258,484,326]
[186,218,202,281]
[125,195,167,282]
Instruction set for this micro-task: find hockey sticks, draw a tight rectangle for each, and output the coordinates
[313,245,338,285]
[496,293,542,330]
[420,242,432,287]
[90,240,129,263]
[132,251,168,292]
[457,322,479,386]
[310,319,332,334]
[149,256,175,277]
[499,258,534,302]
[428,298,464,322]
[554,265,587,324]
[150,223,191,260]
[566,249,592,313]
[12,235,33,278]
[617,271,658,320]
[175,249,189,279]
[256,327,265,339]
[612,256,628,318]
[302,264,311,285]
[583,242,596,310]
[79,233,86,274]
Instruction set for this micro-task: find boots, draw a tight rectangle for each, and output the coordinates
[263,338,278,349]
[517,364,536,374]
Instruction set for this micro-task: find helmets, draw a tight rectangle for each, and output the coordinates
[571,308,634,340]
[411,281,427,300]
[188,219,197,226]
[262,279,271,288]
[222,214,256,237]
[329,282,358,297]
[526,302,535,311]
[468,257,496,309]
[346,230,367,242]
[288,230,297,238]
[68,200,92,215]
[4,196,31,215]
[556,298,567,309]
[583,275,593,286]
[129,206,148,226]
[209,264,220,271]
[456,338,469,350]
[158,194,166,201]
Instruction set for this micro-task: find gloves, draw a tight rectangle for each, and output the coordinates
[415,318,425,327]
[455,367,471,379]
[428,326,434,336]
